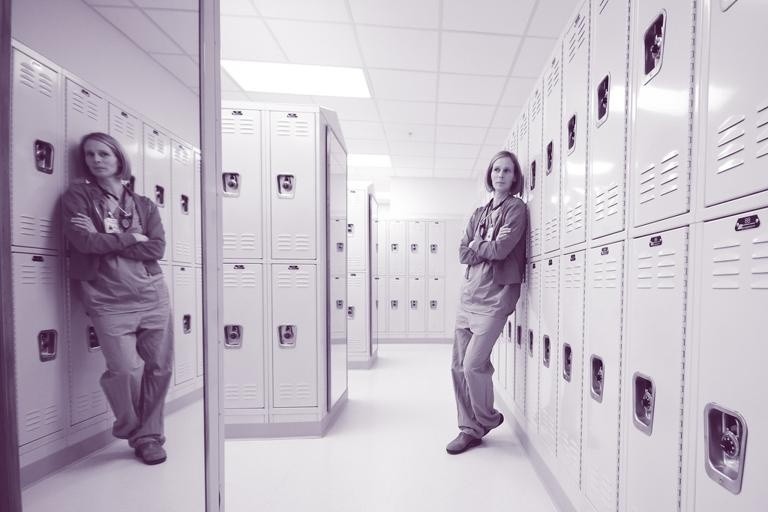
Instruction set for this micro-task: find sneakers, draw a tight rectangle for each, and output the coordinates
[481,414,503,439]
[133,441,167,466]
[445,433,481,454]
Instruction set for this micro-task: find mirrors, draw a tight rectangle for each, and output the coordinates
[0,1,227,512]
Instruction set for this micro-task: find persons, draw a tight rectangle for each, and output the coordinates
[61,130,178,466]
[446,149,531,457]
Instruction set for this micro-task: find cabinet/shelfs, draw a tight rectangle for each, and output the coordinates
[373,217,468,343]
[213,99,347,440]
[346,188,380,369]
[8,37,203,491]
[485,1,768,511]
